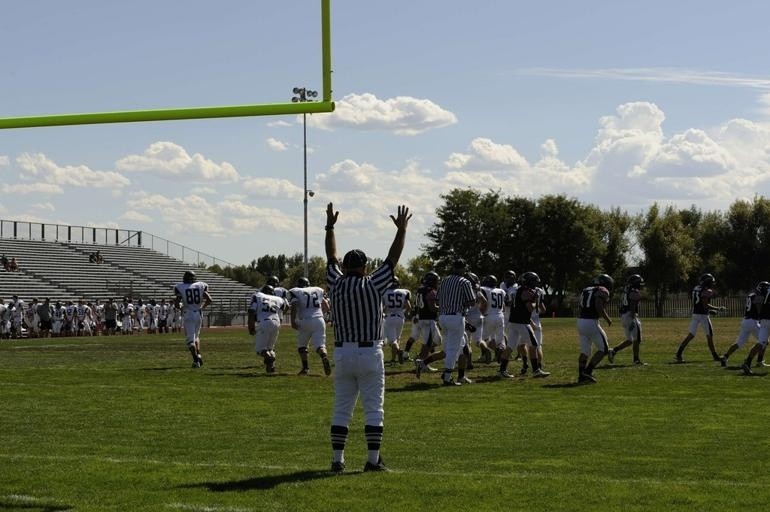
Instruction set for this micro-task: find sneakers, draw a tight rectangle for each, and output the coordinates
[322,355,332,376]
[391,346,553,387]
[755,360,770,369]
[719,354,728,366]
[296,366,311,376]
[607,347,617,363]
[632,360,648,366]
[191,353,204,369]
[330,459,346,473]
[739,363,754,376]
[363,453,392,474]
[576,371,598,386]
[672,354,686,364]
[263,352,276,373]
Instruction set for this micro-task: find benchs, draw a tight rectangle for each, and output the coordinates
[0,237,262,317]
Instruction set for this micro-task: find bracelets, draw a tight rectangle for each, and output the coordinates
[325,225,335,230]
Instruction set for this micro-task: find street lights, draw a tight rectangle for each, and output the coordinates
[291,86,319,278]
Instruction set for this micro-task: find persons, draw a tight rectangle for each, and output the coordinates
[325,200,413,468]
[743,288,770,377]
[674,273,726,364]
[719,281,770,368]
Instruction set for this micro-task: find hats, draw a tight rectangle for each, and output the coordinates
[342,248,372,266]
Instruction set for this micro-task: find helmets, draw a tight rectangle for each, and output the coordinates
[755,280,769,295]
[295,276,311,288]
[518,270,542,289]
[182,269,197,284]
[261,284,276,295]
[699,272,716,288]
[266,275,280,287]
[452,258,469,273]
[392,275,400,289]
[502,269,518,282]
[592,272,615,290]
[483,274,499,287]
[419,269,442,289]
[627,273,646,289]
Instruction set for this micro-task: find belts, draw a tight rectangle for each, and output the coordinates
[257,318,279,323]
[438,310,466,316]
[333,339,383,349]
[182,310,201,313]
[381,313,405,318]
